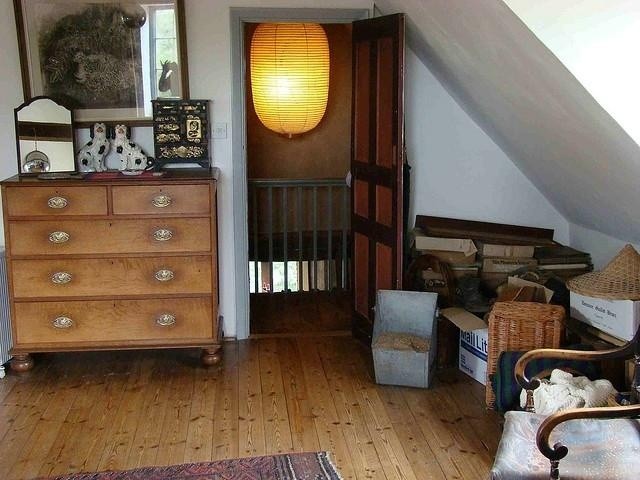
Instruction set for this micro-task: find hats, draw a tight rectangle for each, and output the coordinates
[566,243,639,300]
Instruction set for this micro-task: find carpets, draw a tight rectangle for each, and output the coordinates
[31,452,343,480]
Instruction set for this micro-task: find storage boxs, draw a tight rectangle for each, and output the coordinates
[439,275,555,386]
[569,291,640,342]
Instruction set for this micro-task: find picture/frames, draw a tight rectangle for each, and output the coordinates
[14,0,189,127]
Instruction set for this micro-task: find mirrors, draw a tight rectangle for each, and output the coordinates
[14,95,79,177]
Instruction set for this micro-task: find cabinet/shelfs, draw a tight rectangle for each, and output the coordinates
[0,167,224,373]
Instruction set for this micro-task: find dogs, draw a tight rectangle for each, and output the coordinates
[110,123,155,171]
[77,121,111,173]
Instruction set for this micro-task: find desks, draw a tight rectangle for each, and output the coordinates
[562,320,633,391]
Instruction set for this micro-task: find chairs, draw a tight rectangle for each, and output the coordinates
[513,324,640,480]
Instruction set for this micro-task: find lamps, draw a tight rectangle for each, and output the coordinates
[250,23,331,139]
[23,128,50,172]
